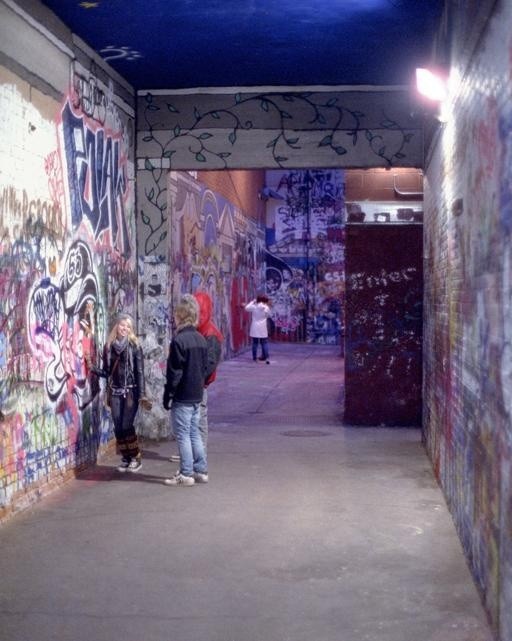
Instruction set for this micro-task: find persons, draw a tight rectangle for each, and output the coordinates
[161,297,209,487]
[169,291,223,464]
[244,295,272,361]
[88,315,148,473]
[240,296,269,360]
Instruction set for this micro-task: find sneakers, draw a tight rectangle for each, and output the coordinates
[193,472,209,484]
[125,455,142,474]
[115,456,130,473]
[163,471,196,488]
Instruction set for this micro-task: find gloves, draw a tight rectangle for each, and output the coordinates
[163,393,172,411]
[138,397,153,411]
